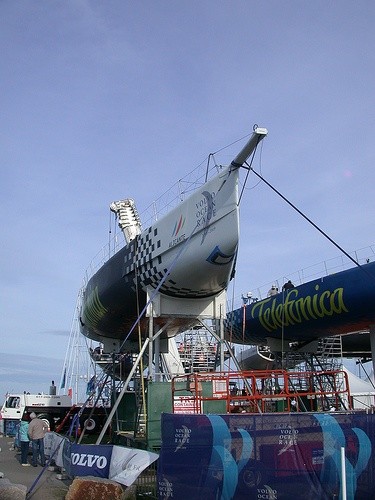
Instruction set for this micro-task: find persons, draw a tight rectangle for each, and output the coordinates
[269,284,278,295]
[282,280,294,292]
[19,413,31,466]
[28,412,48,467]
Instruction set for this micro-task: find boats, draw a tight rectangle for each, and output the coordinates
[76,128,270,354]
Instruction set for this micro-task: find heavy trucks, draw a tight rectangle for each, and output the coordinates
[1,393,114,436]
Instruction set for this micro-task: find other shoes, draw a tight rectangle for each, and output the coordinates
[22,463,31,466]
[34,463,37,467]
[42,463,46,467]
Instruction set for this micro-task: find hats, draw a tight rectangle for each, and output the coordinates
[30,413,36,419]
